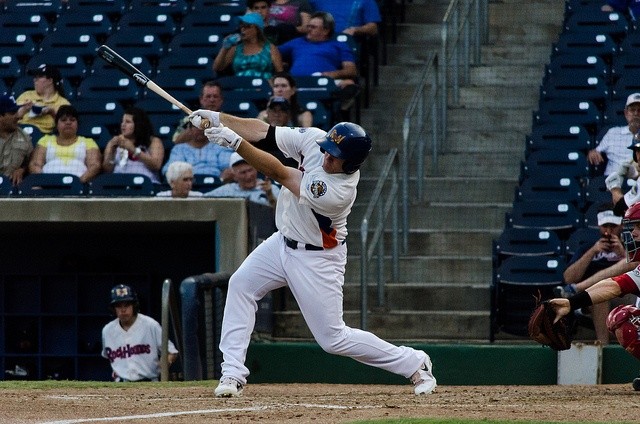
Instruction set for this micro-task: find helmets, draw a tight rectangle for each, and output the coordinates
[622,202,640,263]
[315,122,373,174]
[110,284,140,317]
[627,132,640,162]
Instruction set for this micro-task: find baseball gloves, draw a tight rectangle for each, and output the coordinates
[529,302,573,351]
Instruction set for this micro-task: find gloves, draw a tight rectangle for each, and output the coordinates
[204,123,243,152]
[605,171,625,191]
[188,109,220,131]
[621,163,640,181]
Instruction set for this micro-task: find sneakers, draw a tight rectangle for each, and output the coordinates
[554,283,578,297]
[214,377,244,397]
[633,378,640,390]
[409,350,437,395]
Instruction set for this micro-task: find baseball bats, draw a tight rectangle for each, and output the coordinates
[97,45,210,129]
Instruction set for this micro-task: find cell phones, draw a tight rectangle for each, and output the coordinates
[602,233,612,252]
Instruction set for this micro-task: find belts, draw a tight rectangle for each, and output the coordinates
[284,236,346,250]
[119,377,152,382]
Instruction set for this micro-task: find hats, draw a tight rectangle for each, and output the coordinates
[597,210,623,226]
[0,94,26,112]
[626,92,640,106]
[230,152,244,168]
[27,63,60,78]
[269,98,289,109]
[231,12,264,33]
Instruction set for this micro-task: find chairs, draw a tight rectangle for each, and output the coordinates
[0,174,15,197]
[338,35,361,127]
[296,88,330,130]
[155,174,223,194]
[16,173,87,197]
[256,171,283,190]
[87,173,156,197]
[293,75,338,123]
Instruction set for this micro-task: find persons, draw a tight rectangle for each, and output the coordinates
[543,263,640,392]
[10,62,73,153]
[0,98,35,188]
[185,107,440,396]
[267,0,299,21]
[255,94,298,178]
[588,92,640,193]
[311,0,381,58]
[211,13,283,95]
[163,115,238,174]
[614,139,639,216]
[103,106,166,195]
[29,105,102,195]
[99,284,180,382]
[270,10,356,104]
[173,83,226,144]
[563,210,640,345]
[208,149,282,237]
[255,74,313,128]
[156,160,204,196]
[249,0,285,31]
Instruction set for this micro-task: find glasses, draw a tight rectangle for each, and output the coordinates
[306,24,317,29]
[253,6,268,10]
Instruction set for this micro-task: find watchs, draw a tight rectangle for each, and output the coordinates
[131,146,141,159]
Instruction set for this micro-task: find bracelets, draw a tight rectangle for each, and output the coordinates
[566,291,594,315]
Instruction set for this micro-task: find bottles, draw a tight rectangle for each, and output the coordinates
[223,33,245,49]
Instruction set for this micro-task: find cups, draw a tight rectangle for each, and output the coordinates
[30,102,48,114]
